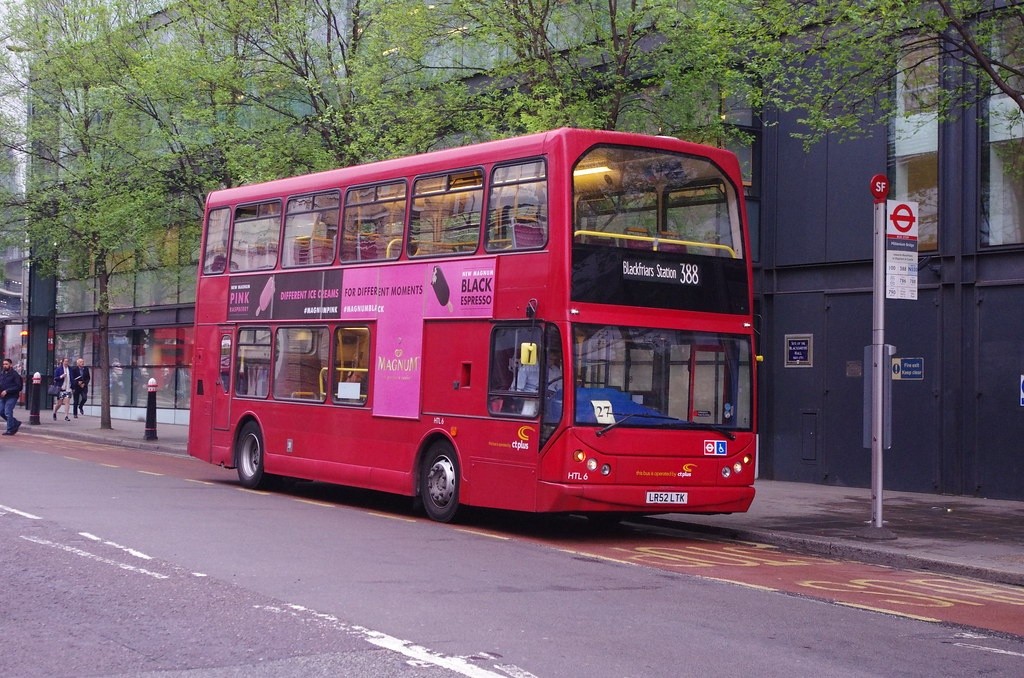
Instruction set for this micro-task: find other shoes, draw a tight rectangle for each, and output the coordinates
[13,421,22,433]
[65,417,70,421]
[2,426,16,435]
[74,414,78,418]
[79,407,85,416]
[52,414,56,421]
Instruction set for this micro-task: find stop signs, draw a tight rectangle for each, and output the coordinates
[887,199,918,240]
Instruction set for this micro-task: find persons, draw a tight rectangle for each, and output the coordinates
[53,358,75,421]
[121,360,142,406]
[72,358,90,419]
[0,359,23,436]
[162,363,170,388]
[110,357,122,405]
[174,360,191,408]
[508,349,562,392]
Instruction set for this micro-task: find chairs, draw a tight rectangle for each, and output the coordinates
[291,392,317,400]
[587,227,687,252]
[215,215,547,270]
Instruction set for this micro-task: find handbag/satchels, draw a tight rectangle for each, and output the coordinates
[48,377,60,397]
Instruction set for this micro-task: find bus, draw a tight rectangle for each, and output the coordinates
[188,128,766,522]
